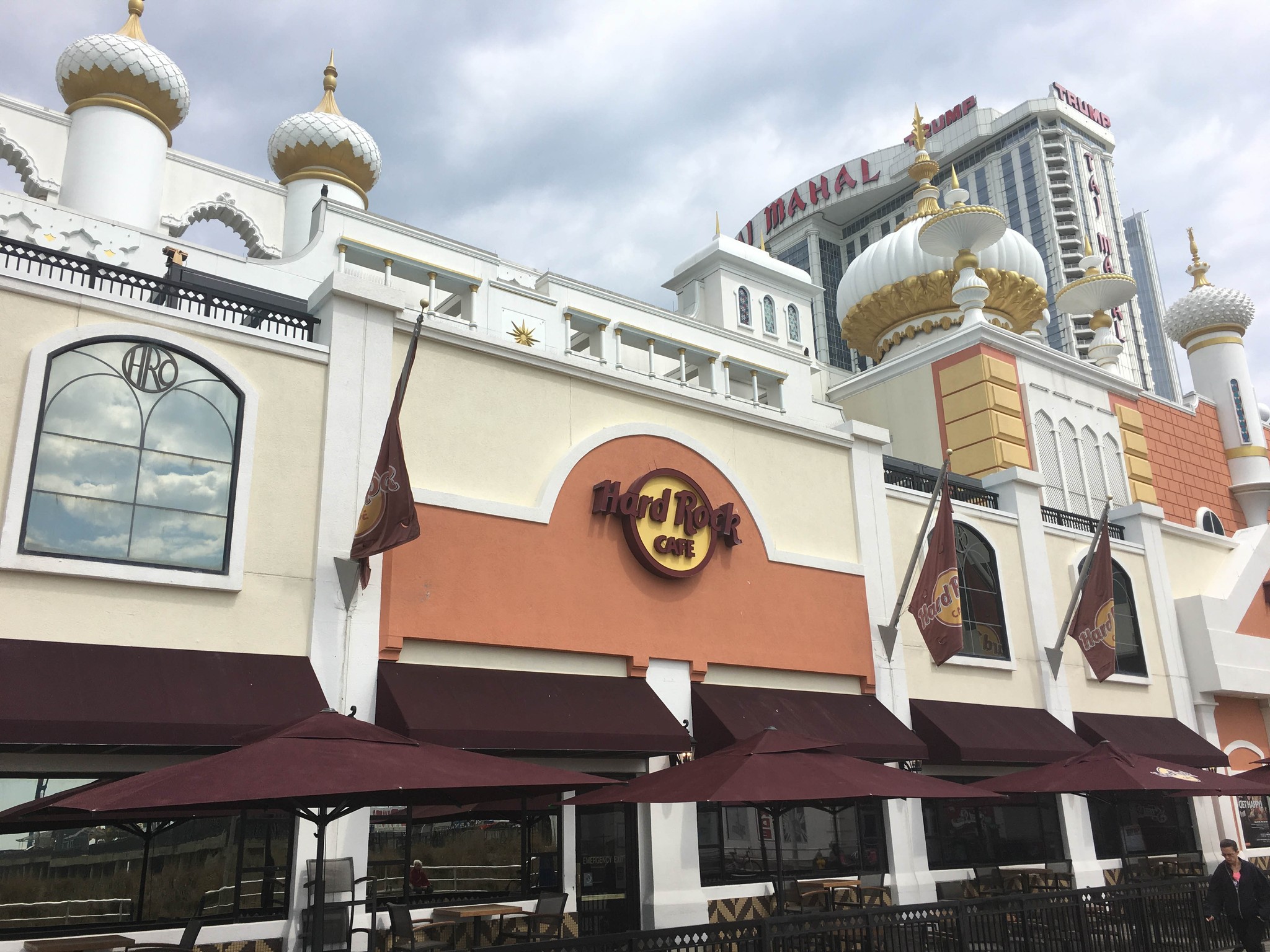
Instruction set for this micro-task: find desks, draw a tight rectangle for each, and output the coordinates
[24,935,135,952]
[1140,857,1191,879]
[995,868,1053,893]
[797,880,862,912]
[434,903,523,947]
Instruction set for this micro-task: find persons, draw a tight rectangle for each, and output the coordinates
[1203,839,1270,952]
[410,860,433,902]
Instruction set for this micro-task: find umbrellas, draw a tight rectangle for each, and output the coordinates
[0,778,1168,923]
[18,707,1270,952]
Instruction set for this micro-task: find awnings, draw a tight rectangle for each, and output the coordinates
[908,698,1093,767]
[0,639,329,755]
[374,660,691,760]
[1072,711,1231,769]
[691,682,928,765]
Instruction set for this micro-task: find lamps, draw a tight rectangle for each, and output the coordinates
[669,719,696,766]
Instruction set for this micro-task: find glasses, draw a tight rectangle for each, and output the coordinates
[1221,851,1236,856]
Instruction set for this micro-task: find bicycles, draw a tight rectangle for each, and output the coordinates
[724,847,763,877]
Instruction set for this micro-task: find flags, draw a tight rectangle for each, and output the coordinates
[1067,515,1115,683]
[349,338,419,591]
[908,473,963,667]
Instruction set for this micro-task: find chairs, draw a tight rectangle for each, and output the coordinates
[125,919,203,952]
[972,859,1072,897]
[1125,850,1203,888]
[298,857,377,952]
[387,889,568,952]
[772,871,885,916]
